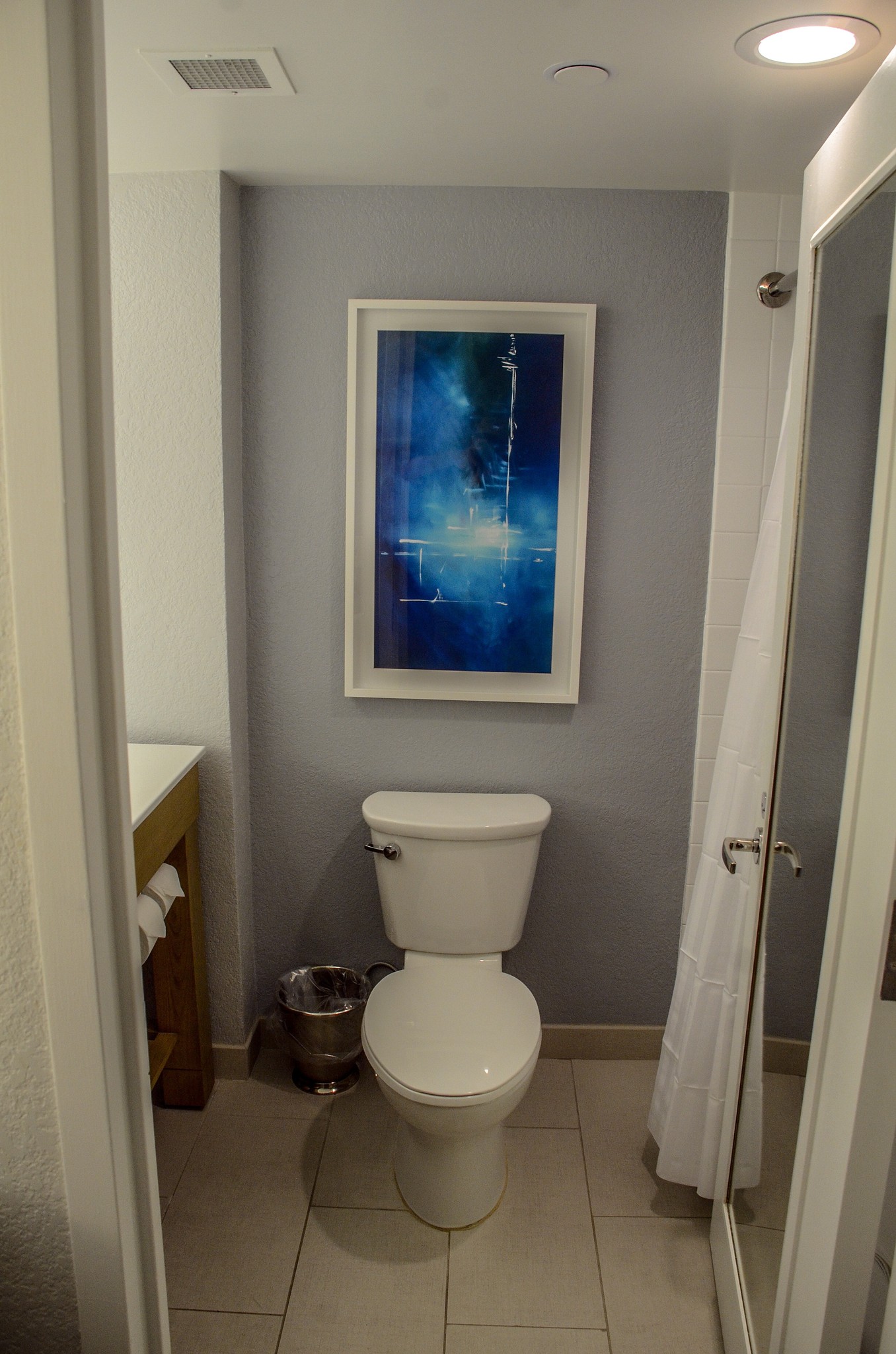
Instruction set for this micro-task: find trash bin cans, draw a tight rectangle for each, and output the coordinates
[273,964,372,1096]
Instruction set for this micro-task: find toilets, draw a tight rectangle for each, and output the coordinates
[358,792,552,1232]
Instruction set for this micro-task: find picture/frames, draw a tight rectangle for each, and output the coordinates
[342,295,599,711]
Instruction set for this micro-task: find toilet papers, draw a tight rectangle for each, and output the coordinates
[134,894,167,965]
[142,863,187,920]
